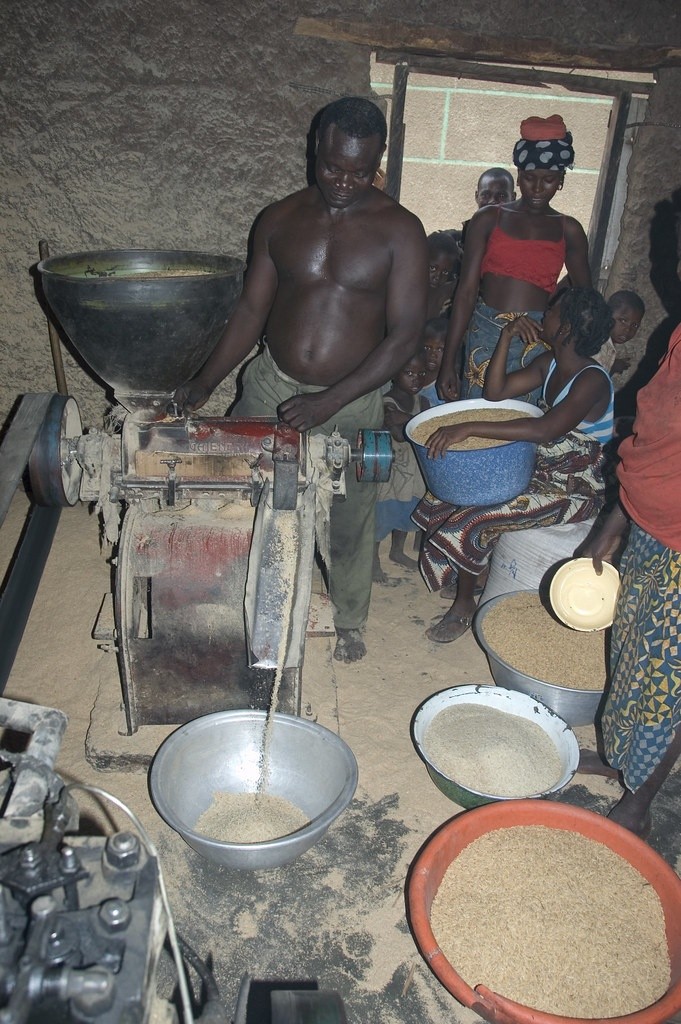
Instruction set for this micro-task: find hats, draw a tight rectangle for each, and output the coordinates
[513,114,574,171]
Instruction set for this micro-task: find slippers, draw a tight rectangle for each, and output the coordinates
[426,611,473,643]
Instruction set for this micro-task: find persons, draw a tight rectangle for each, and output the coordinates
[167,97,428,664]
[410,287,614,644]
[372,229,467,584]
[475,167,516,209]
[590,290,645,378]
[434,114,591,407]
[581,190,681,842]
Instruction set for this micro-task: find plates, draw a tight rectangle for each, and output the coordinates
[549,556,624,633]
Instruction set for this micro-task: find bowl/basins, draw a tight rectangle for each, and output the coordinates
[148,709,359,867]
[473,588,611,727]
[403,398,545,506]
[411,684,581,810]
[407,801,681,1024]
